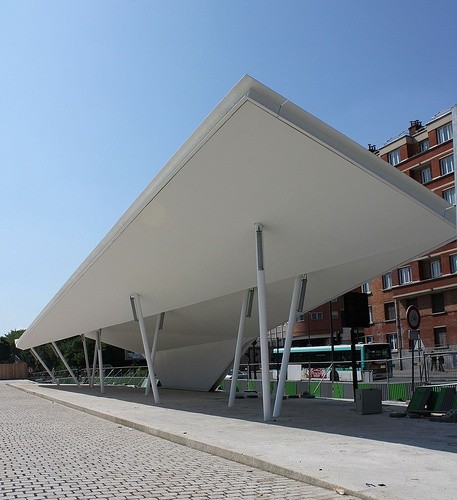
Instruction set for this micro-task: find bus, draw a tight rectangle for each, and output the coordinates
[271,343,392,381]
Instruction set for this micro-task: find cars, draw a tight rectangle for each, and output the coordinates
[224,369,248,379]
